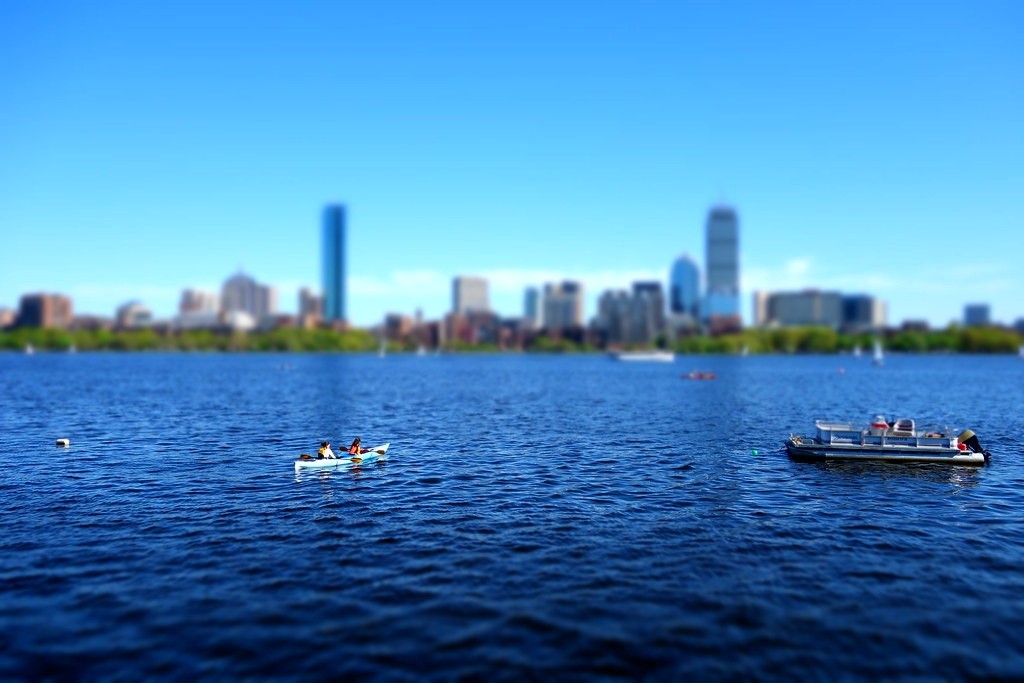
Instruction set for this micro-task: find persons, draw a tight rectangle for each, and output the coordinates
[318,442,336,459]
[349,437,361,454]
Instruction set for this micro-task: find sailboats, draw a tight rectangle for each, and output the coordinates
[873,340,885,366]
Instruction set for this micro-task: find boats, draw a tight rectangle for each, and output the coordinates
[609,350,675,363]
[294,443,392,473]
[680,373,716,380]
[785,421,989,464]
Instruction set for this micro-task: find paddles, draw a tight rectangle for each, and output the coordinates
[301,454,362,463]
[340,446,385,454]
